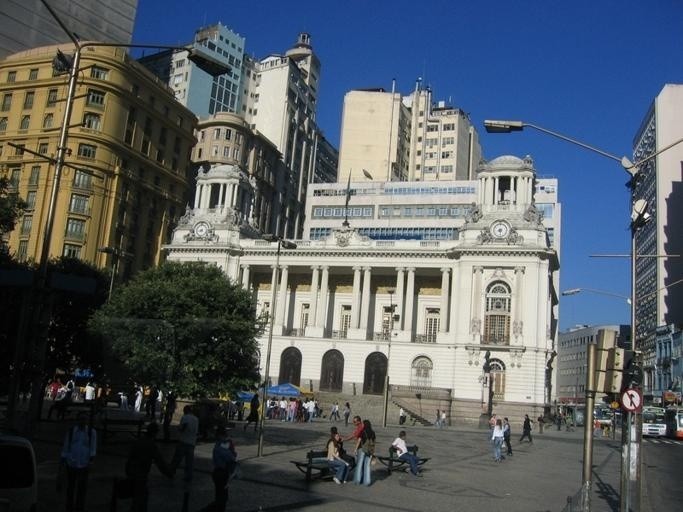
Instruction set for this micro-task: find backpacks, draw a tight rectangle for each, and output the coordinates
[361,431,375,455]
[270,402,275,406]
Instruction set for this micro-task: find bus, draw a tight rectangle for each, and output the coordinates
[667,407,683,439]
[641,406,667,438]
[556,402,612,427]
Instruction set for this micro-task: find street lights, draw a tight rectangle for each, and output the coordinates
[255,234,298,457]
[97,245,136,302]
[381,290,396,428]
[482,116,653,512]
[39,41,235,263]
[560,271,683,350]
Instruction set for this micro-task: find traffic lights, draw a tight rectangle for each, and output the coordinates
[623,350,639,394]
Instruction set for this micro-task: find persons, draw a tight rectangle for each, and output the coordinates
[439,410,450,430]
[558,414,562,431]
[392,431,423,477]
[435,409,440,427]
[593,418,601,438]
[565,413,572,433]
[39,376,374,510]
[489,413,497,437]
[519,414,534,442]
[537,415,547,435]
[399,407,404,425]
[503,418,513,456]
[491,419,505,463]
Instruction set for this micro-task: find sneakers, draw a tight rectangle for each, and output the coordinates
[333,477,341,484]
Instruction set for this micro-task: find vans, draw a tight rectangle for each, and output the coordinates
[0,434,39,512]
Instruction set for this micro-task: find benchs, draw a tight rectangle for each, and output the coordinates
[378,445,429,470]
[101,418,147,445]
[289,449,357,479]
